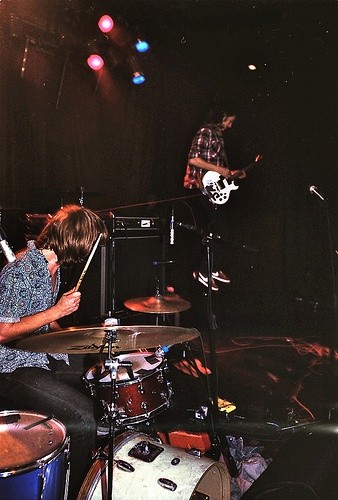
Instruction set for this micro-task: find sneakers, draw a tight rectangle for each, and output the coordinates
[194,271,219,291]
[210,270,231,284]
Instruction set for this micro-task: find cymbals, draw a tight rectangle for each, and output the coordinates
[123,294,195,314]
[14,323,204,355]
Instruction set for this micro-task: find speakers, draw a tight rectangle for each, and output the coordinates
[105,234,164,316]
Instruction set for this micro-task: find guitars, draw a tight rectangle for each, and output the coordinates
[198,150,263,207]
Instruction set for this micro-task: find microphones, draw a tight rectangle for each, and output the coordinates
[154,345,175,357]
[169,208,176,245]
[310,186,326,201]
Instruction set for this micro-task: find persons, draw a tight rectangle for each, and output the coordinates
[0,205,109,500]
[183,106,246,291]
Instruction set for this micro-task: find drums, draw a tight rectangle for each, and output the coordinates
[1,411,71,500]
[78,427,234,500]
[82,349,175,428]
